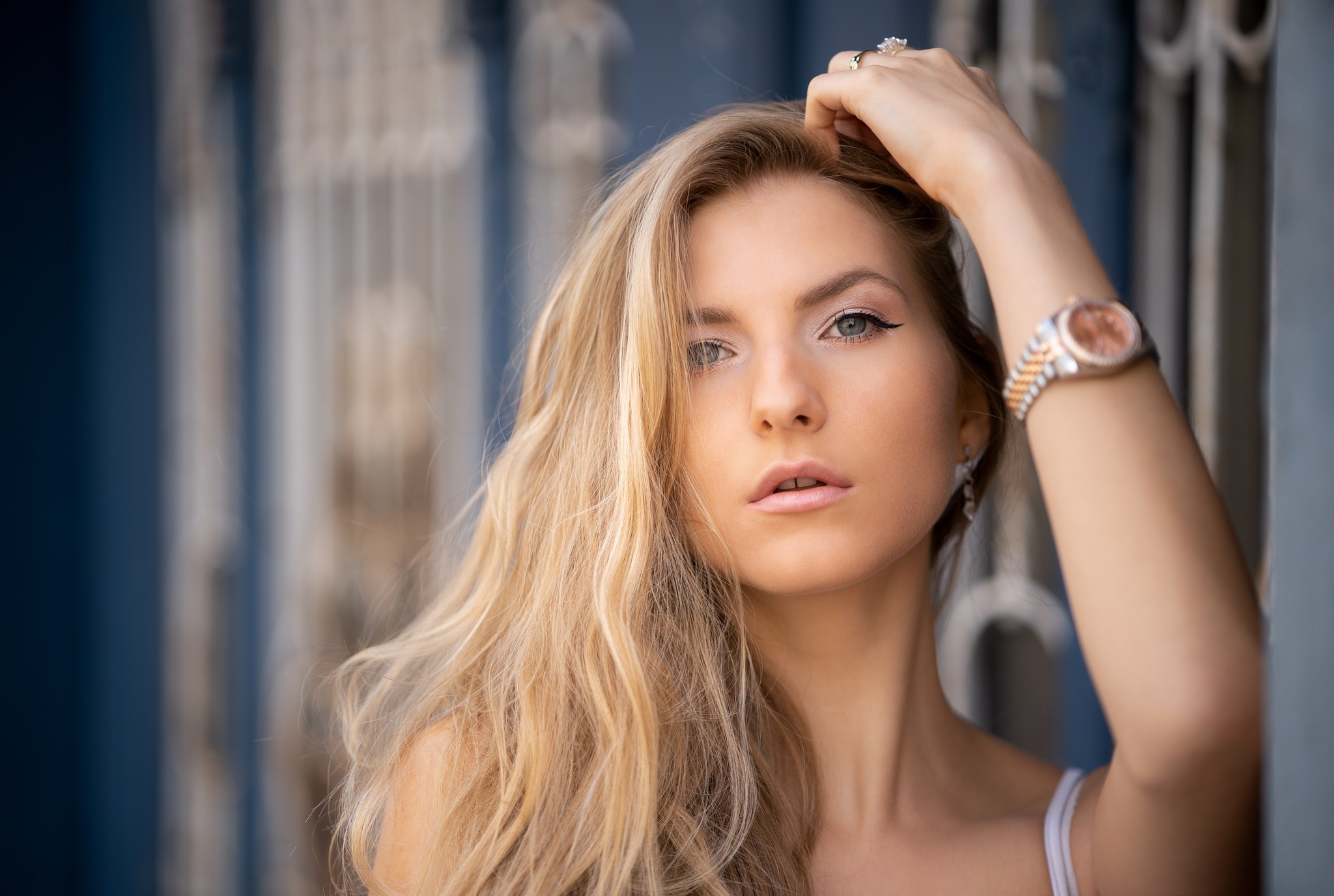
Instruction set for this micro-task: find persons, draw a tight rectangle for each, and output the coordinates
[311,36,1264,896]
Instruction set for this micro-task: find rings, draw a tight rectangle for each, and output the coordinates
[876,37,907,56]
[850,50,877,70]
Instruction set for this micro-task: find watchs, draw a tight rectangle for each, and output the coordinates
[1001,299,1162,421]
